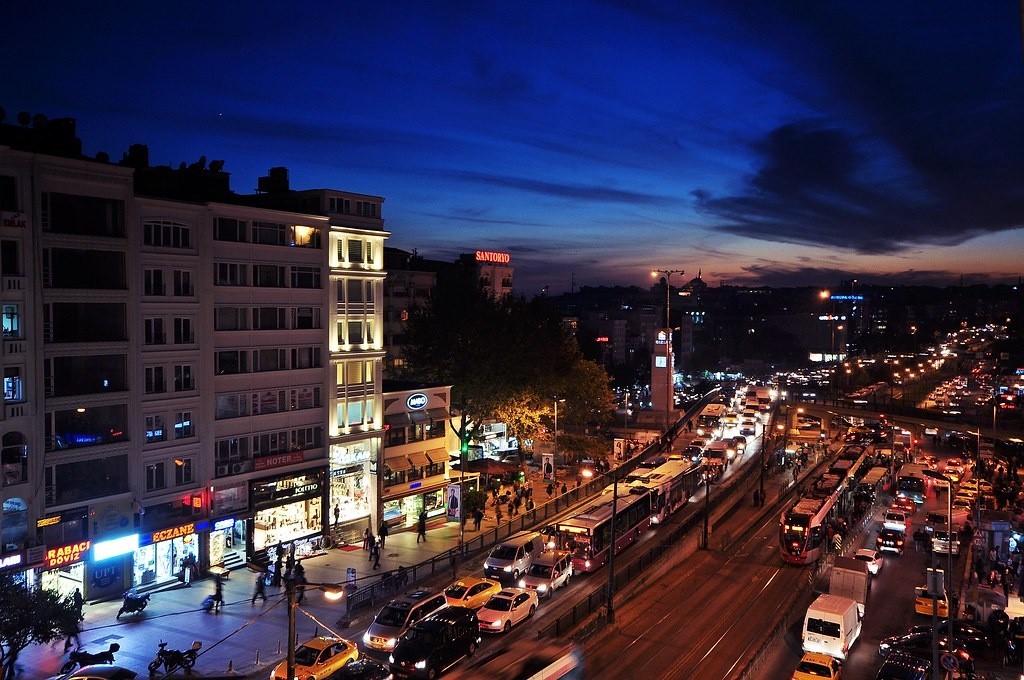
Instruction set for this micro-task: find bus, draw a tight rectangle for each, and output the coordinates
[779,444,870,569]
[545,485,658,574]
[627,459,698,529]
[858,466,888,501]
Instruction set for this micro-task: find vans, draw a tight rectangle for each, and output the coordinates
[518,549,573,599]
[359,585,447,653]
[800,592,864,664]
[389,606,480,680]
[483,530,547,581]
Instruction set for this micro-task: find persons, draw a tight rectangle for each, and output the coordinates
[823,487,870,550]
[333,503,341,529]
[417,512,427,543]
[596,457,619,473]
[791,450,816,481]
[546,473,582,499]
[544,456,553,480]
[362,519,388,570]
[614,442,623,460]
[212,540,306,617]
[976,463,1024,597]
[73,588,85,612]
[627,439,655,459]
[448,488,459,517]
[666,437,674,452]
[753,488,768,506]
[912,528,930,553]
[461,489,501,531]
[506,487,535,520]
[686,419,693,432]
[1009,616,1023,635]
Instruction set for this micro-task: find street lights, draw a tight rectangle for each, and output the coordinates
[967,428,982,528]
[784,407,797,456]
[286,572,345,680]
[593,465,650,625]
[759,424,776,499]
[703,448,724,550]
[922,468,954,680]
[554,394,567,443]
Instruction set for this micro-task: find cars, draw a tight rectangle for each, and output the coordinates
[832,416,902,447]
[44,665,147,680]
[853,462,998,580]
[269,635,359,680]
[789,652,842,679]
[444,577,503,612]
[914,590,950,619]
[476,587,539,633]
[334,658,395,680]
[928,375,995,409]
[600,378,772,499]
[875,621,994,680]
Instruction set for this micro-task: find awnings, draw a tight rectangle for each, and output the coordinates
[385,408,452,428]
[384,449,451,473]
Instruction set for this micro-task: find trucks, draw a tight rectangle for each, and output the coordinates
[829,555,873,625]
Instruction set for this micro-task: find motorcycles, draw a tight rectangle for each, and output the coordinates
[147,638,202,672]
[60,641,121,674]
[116,588,150,618]
